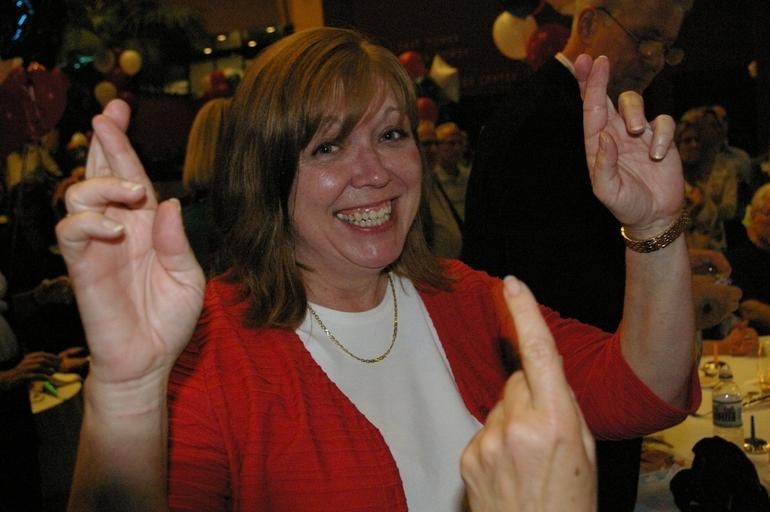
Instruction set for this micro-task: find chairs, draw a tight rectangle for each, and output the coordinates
[667,438,770,512]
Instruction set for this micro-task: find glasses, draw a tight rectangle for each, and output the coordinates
[597,7,685,66]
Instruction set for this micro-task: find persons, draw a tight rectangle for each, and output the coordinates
[454,271,602,509]
[0,0,770,430]
[51,21,704,510]
[453,0,693,510]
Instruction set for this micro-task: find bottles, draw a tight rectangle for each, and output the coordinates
[708,369,748,449]
